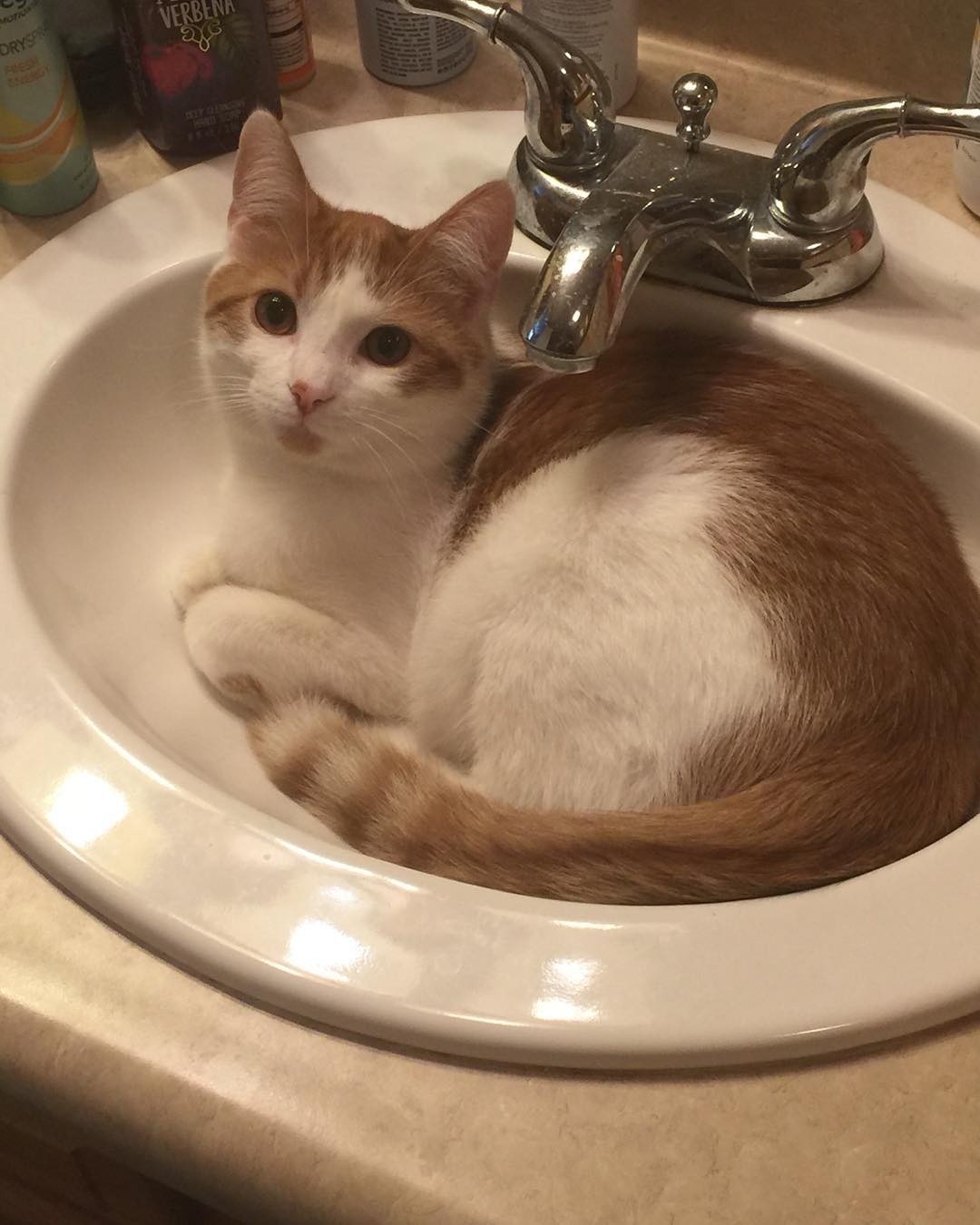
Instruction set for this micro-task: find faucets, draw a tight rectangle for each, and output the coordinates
[404,0,978,378]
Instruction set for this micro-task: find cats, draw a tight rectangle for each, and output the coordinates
[171,108,980,906]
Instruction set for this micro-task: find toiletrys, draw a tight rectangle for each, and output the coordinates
[0,2,319,219]
[354,3,478,89]
[522,1,643,109]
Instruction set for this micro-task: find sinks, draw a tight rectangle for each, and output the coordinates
[0,109,980,1076]
